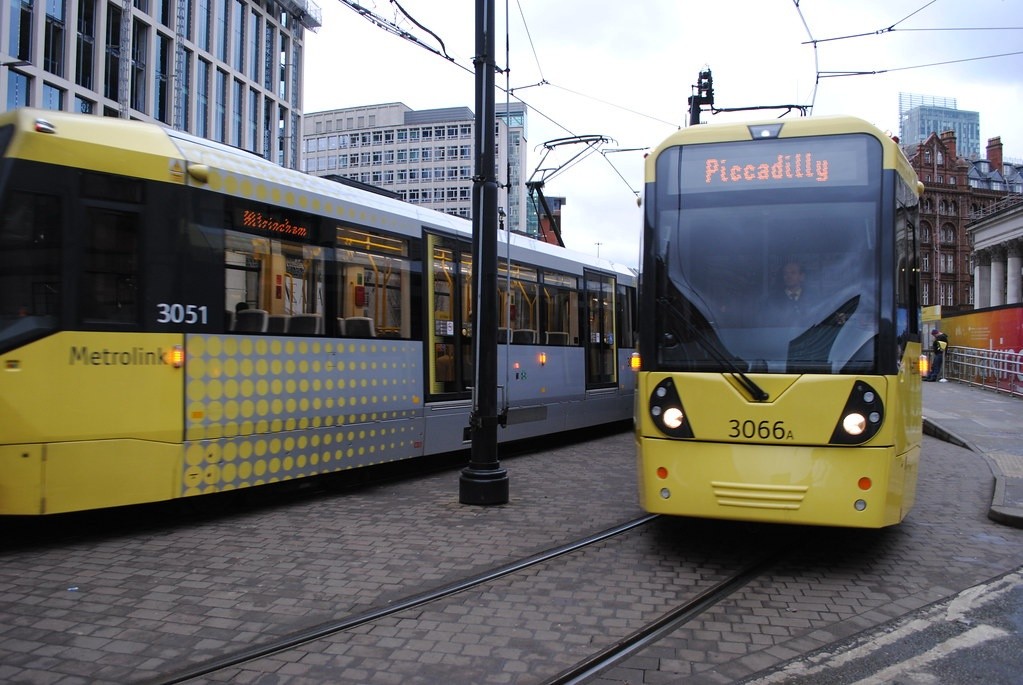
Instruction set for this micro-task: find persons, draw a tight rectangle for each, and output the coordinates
[763,260,819,330]
[922,329,948,382]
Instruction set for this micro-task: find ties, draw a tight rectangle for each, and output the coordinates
[791,293,797,302]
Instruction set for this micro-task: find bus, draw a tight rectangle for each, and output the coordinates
[1,109,642,528]
[634,114,922,528]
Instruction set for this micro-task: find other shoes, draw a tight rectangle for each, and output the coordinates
[927,377,936,381]
